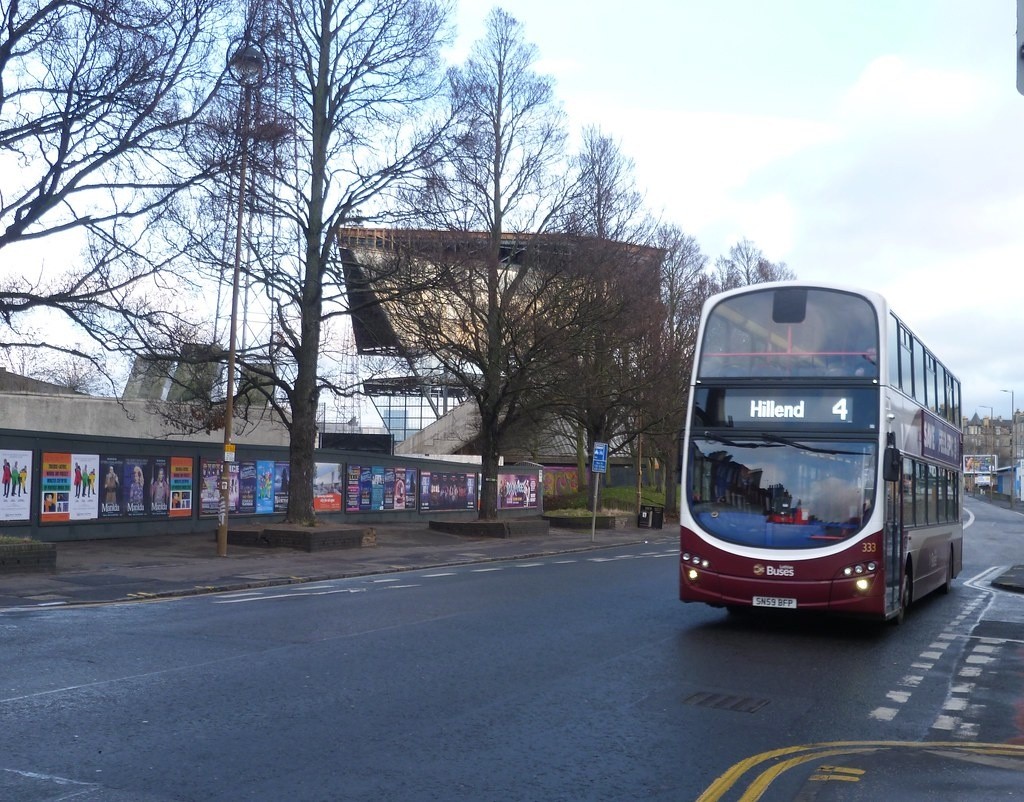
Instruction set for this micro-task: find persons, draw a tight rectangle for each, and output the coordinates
[172,492,190,508]
[129,467,144,506]
[152,468,170,505]
[397,478,405,498]
[429,473,468,509]
[317,482,341,495]
[44,493,68,512]
[499,480,507,508]
[514,479,531,507]
[74,462,96,498]
[808,460,861,523]
[104,464,119,505]
[2,459,28,498]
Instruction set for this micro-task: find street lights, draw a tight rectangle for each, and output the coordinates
[217,25,270,557]
[980,405,993,504]
[1002,390,1015,511]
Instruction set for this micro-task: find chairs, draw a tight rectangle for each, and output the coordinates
[717,360,874,376]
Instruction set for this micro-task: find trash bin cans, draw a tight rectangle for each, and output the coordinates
[979,486,986,495]
[638,502,665,530]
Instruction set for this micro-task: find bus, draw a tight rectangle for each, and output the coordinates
[678,280,964,624]
